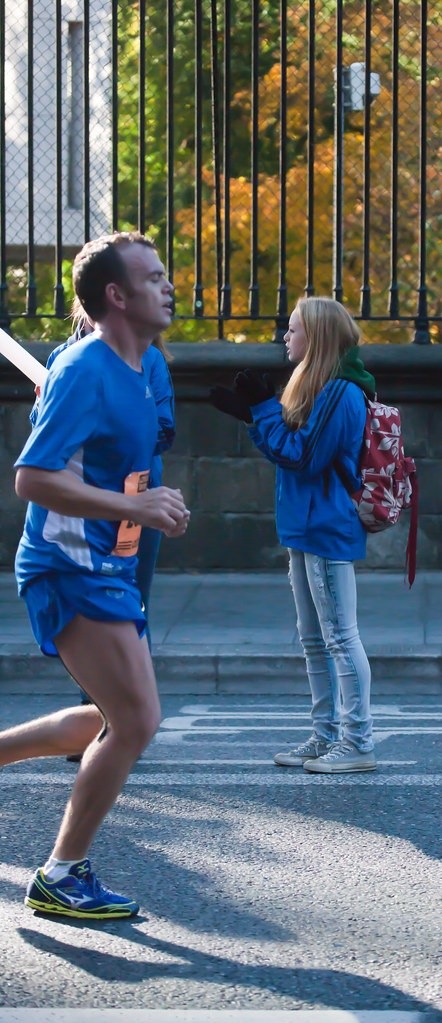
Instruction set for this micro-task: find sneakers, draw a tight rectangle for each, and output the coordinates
[24,857,139,918]
[304,739,377,773]
[273,731,334,766]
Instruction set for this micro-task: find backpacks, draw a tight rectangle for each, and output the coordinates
[314,374,418,588]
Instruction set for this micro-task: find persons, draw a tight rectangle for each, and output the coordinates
[208,297,377,773]
[30,296,176,763]
[0,233,191,919]
